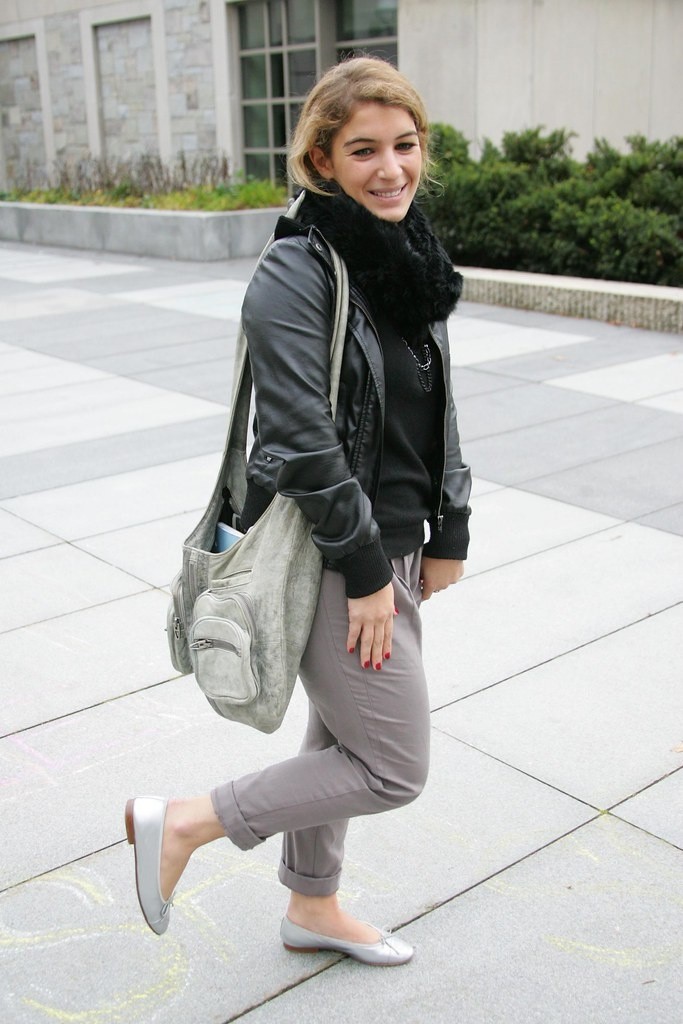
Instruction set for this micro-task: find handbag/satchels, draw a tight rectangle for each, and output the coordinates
[166,229,351,734]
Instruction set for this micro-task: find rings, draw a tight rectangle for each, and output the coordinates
[434,591,439,593]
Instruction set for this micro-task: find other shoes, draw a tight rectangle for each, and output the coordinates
[280,914,414,966]
[125,797,176,935]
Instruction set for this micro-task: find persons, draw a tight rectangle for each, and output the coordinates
[124,57,471,969]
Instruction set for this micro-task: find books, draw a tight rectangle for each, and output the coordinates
[214,521,245,555]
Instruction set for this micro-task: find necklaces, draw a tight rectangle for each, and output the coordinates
[400,333,434,395]
